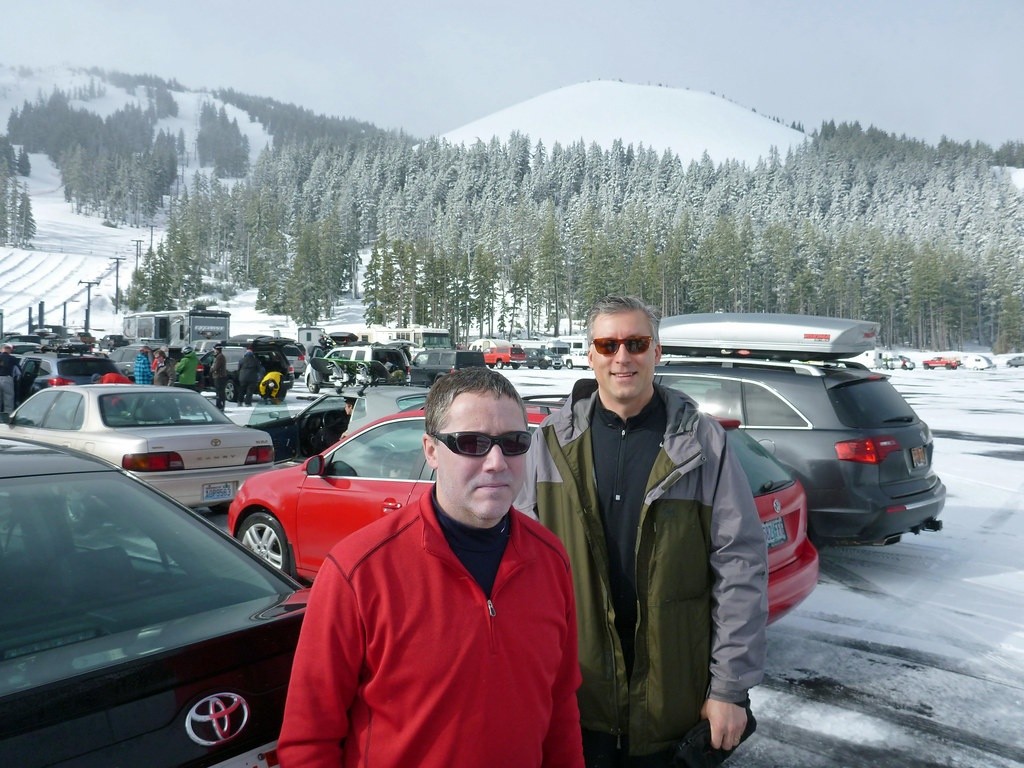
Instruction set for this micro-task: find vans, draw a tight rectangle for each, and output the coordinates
[960,354,993,370]
[887,356,915,371]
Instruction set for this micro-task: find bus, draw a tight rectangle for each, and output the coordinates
[123,304,231,349]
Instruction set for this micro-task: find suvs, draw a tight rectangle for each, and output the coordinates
[646,312,949,551]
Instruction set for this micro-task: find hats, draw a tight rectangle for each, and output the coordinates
[344,399,357,404]
[214,344,223,351]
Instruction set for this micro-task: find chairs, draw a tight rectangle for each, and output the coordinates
[703,388,732,417]
[22,545,137,605]
[135,404,168,424]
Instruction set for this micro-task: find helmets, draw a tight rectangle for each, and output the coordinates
[139,345,152,356]
[181,346,194,355]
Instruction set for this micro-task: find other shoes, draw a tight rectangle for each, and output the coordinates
[237,403,242,407]
[244,403,252,407]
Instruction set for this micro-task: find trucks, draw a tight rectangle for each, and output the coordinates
[859,349,888,369]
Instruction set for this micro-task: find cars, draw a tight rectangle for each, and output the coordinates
[0,434,312,768]
[0,325,592,408]
[0,383,274,514]
[228,397,819,629]
[242,384,431,469]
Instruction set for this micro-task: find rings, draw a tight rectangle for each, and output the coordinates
[733,736,741,741]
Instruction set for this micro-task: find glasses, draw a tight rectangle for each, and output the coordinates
[590,336,654,355]
[431,431,532,456]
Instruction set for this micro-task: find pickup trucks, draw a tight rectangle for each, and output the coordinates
[1006,355,1024,367]
[922,356,961,370]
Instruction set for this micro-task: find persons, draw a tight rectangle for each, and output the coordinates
[259,371,286,404]
[134,345,153,385]
[173,346,200,416]
[91,373,135,404]
[211,344,227,412]
[0,344,22,414]
[153,350,176,387]
[506,294,769,768]
[275,367,584,768]
[238,350,258,407]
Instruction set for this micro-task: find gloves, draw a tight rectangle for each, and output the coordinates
[673,706,757,768]
